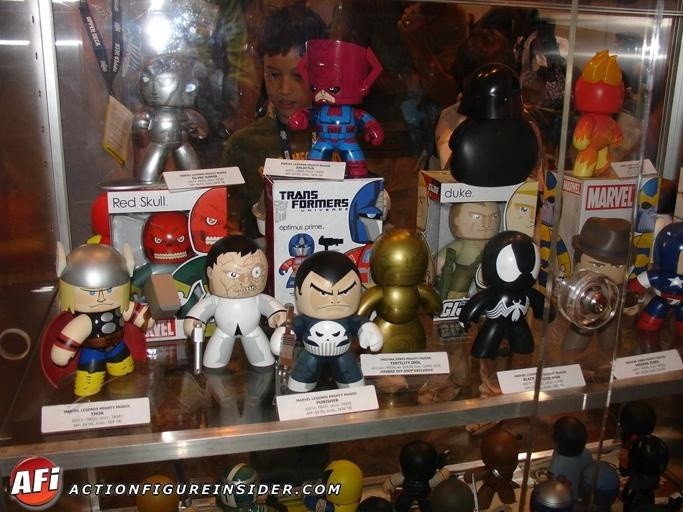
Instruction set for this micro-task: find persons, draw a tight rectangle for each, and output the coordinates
[195,0,264,138]
[397,0,581,176]
[221,6,330,237]
[51,39,683,512]
